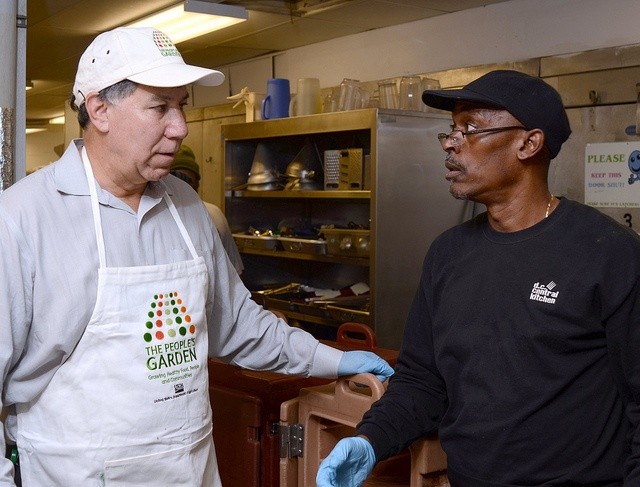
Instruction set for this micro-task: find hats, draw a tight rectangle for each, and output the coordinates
[72,26,224,107]
[422,70,572,156]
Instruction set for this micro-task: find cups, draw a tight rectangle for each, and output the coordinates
[377,79,399,112]
[288,76,321,120]
[398,75,421,112]
[338,77,360,112]
[261,78,290,118]
[419,77,442,113]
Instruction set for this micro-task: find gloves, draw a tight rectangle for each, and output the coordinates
[339,350,394,384]
[316,437,377,487]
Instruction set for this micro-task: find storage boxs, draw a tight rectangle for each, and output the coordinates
[277,236,325,256]
[322,225,371,258]
[233,231,277,250]
[219,107,475,351]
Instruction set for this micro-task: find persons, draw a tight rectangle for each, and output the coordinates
[0,21,392,487]
[314,67,639,487]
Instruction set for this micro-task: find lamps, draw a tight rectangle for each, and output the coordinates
[122,0,248,45]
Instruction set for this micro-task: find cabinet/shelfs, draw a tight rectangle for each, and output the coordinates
[202,323,401,487]
[280,373,450,487]
[173,101,246,214]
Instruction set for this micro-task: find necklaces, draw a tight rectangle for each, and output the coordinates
[543,191,557,219]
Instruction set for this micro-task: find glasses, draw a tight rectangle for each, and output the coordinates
[438,126,528,146]
